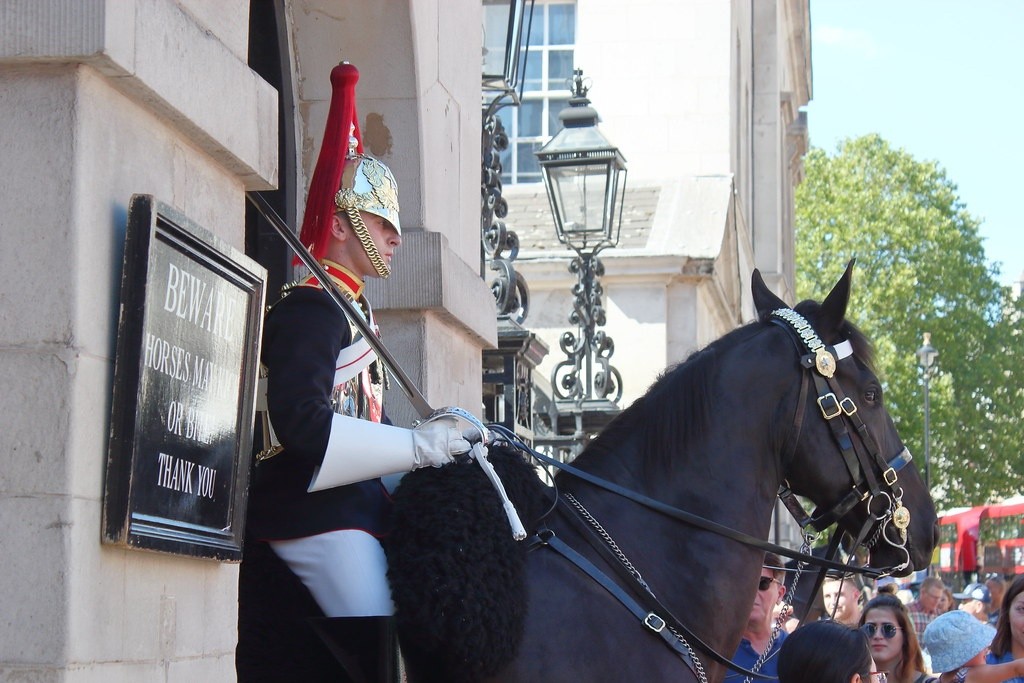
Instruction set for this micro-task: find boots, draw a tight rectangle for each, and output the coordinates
[311,608,407,683]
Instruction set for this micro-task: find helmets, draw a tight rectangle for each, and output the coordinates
[291,61,403,266]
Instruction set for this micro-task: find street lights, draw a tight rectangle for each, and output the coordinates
[533,67,630,460]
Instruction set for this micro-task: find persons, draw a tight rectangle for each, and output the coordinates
[247,152,496,682]
[725,551,1024,683]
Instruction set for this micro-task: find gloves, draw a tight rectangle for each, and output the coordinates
[461,423,504,448]
[307,411,472,492]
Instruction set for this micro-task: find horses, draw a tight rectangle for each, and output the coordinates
[235,254,941,683]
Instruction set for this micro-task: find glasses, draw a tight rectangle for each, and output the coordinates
[758,575,783,591]
[860,622,902,639]
[860,666,890,683]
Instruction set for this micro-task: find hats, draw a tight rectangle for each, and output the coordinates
[924,610,997,673]
[952,584,992,604]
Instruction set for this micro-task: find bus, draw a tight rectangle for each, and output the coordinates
[973,496,1024,586]
[926,504,987,589]
